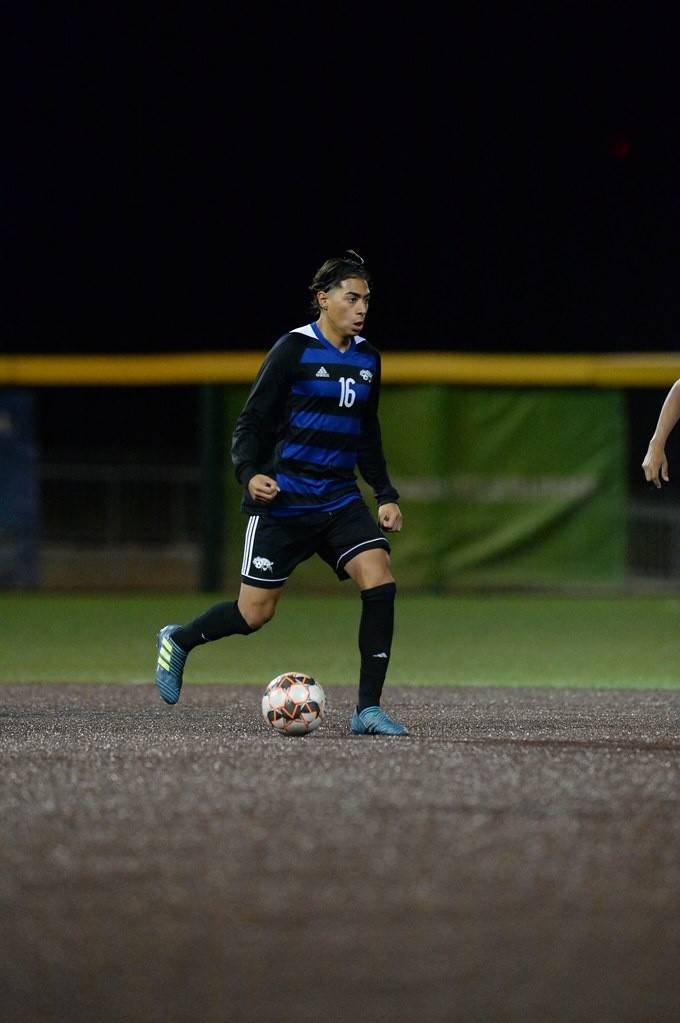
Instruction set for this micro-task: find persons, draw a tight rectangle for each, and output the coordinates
[155,256,412,736]
[642,378,680,489]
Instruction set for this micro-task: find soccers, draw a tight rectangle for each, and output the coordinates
[261,673,329,737]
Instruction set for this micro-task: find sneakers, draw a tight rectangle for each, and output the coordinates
[154,625,188,704]
[351,705,409,736]
